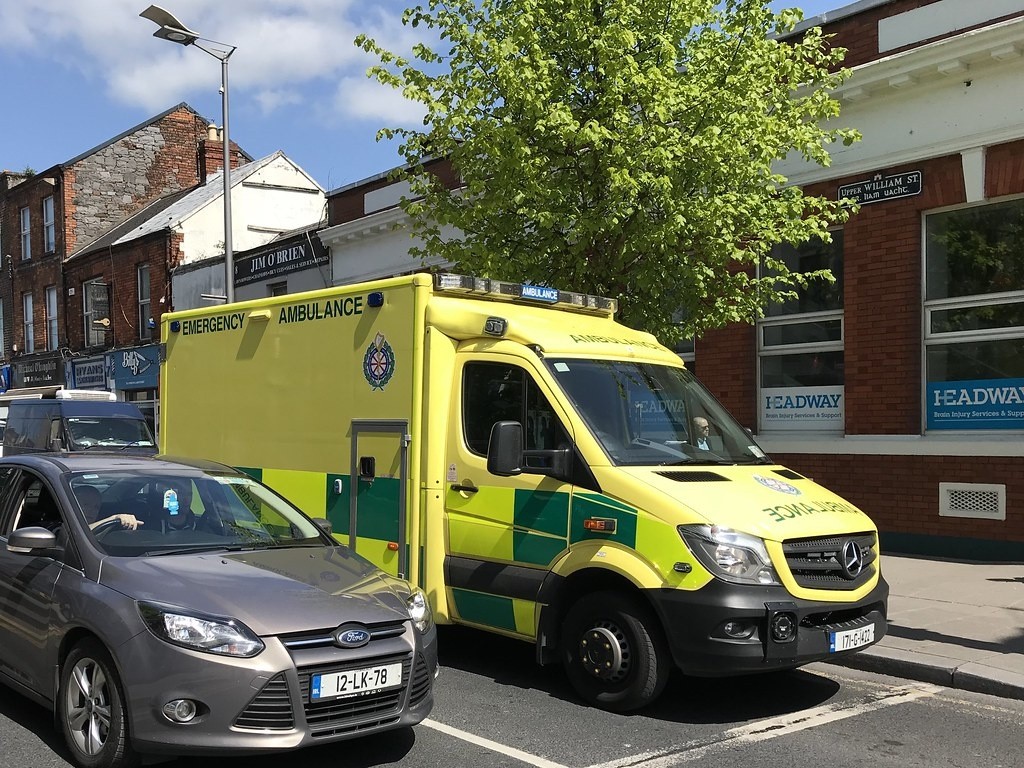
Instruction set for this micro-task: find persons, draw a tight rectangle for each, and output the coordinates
[687,416,714,451]
[43,484,144,552]
[144,476,215,535]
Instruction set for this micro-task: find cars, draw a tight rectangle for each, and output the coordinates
[0,449,440,768]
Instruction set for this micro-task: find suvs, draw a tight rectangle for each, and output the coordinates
[3,399,159,454]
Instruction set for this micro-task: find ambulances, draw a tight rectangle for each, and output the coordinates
[158,273,890,718]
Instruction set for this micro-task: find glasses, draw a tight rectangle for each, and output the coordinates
[701,425,709,431]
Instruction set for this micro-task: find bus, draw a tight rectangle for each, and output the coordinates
[0,385,117,457]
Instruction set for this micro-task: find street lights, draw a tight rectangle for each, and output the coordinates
[140,5,239,305]
